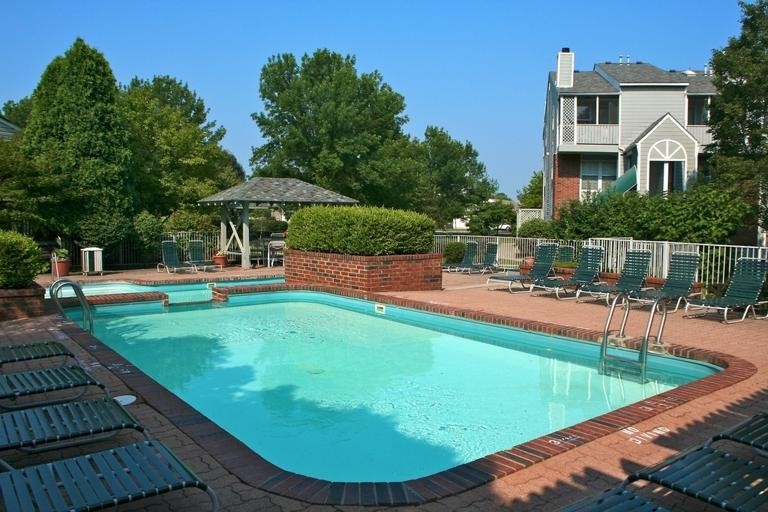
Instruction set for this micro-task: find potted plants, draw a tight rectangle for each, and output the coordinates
[212,251,226,267]
[51,249,72,276]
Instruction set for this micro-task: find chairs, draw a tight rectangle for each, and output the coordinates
[484,243,561,293]
[529,243,606,301]
[157,240,224,274]
[456,242,502,276]
[683,256,768,325]
[442,242,478,275]
[575,248,655,307]
[623,250,703,313]
[542,412,767,512]
[0,341,219,511]
